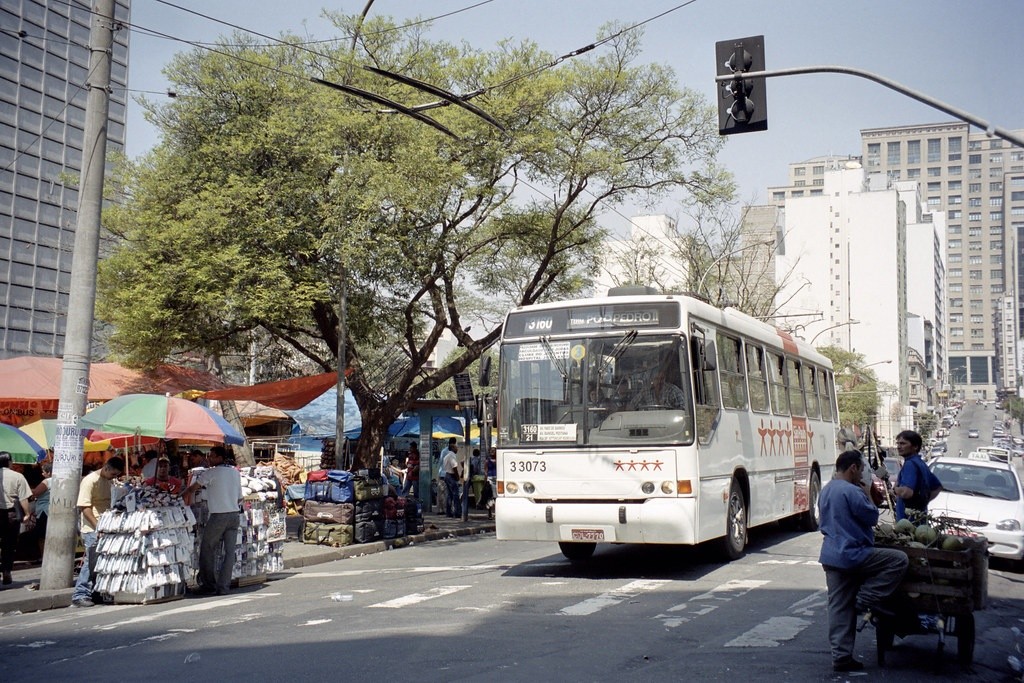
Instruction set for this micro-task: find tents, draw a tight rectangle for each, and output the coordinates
[0,357,236,426]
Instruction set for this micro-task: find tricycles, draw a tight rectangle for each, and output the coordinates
[868,519,994,666]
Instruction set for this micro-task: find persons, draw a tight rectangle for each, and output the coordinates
[954,420,960,426]
[892,430,943,528]
[183,445,244,595]
[959,448,962,457]
[0,451,33,585]
[140,449,211,495]
[818,450,909,672]
[587,367,685,428]
[72,456,125,605]
[31,464,52,565]
[386,436,497,519]
[995,412,997,420]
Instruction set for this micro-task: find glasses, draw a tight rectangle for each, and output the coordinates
[112,473,119,477]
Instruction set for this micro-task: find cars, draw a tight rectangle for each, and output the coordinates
[976,398,1005,409]
[921,452,1024,566]
[993,413,1024,457]
[865,455,903,508]
[919,400,966,461]
[968,428,979,438]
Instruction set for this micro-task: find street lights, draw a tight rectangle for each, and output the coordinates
[940,367,958,418]
[959,372,973,400]
[699,237,892,377]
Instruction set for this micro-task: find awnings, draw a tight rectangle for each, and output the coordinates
[191,366,403,437]
[388,415,498,439]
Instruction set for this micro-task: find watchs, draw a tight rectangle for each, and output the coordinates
[892,487,896,495]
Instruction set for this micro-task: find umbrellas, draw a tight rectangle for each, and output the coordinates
[81,391,244,486]
[0,419,161,488]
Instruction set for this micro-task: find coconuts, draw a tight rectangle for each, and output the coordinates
[879,517,964,552]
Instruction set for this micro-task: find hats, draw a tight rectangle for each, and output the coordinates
[159,453,170,463]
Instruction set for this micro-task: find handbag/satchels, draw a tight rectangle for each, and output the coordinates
[14,498,25,522]
[303,467,424,547]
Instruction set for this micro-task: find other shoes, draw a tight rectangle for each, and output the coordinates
[73,599,94,606]
[834,655,862,671]
[856,599,894,617]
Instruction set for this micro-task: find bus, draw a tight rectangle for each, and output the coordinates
[976,446,1010,464]
[479,283,842,563]
